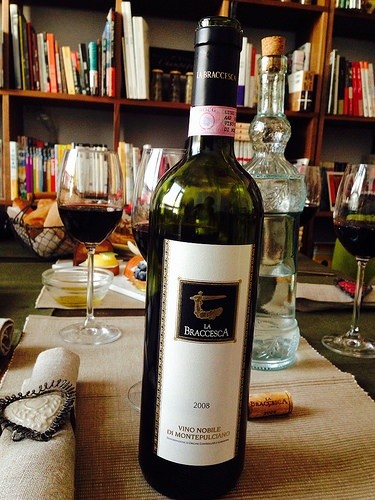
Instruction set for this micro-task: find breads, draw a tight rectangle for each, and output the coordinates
[12,193,87,238]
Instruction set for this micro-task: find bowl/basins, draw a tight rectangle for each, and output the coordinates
[41,267,113,308]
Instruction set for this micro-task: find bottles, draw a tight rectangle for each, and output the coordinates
[138,16,264,500]
[240,36,306,371]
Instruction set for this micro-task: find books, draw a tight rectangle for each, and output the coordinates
[0,0,375,308]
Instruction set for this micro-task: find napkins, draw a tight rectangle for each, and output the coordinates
[0,347,80,500]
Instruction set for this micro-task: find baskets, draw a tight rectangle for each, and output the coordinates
[9,193,76,259]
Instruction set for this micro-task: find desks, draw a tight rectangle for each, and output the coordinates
[0,229,375,499]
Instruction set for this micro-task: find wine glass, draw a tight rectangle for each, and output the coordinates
[54,146,128,346]
[321,161,375,361]
[131,147,186,281]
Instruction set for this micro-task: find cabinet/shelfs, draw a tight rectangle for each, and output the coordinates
[0,0,375,217]
